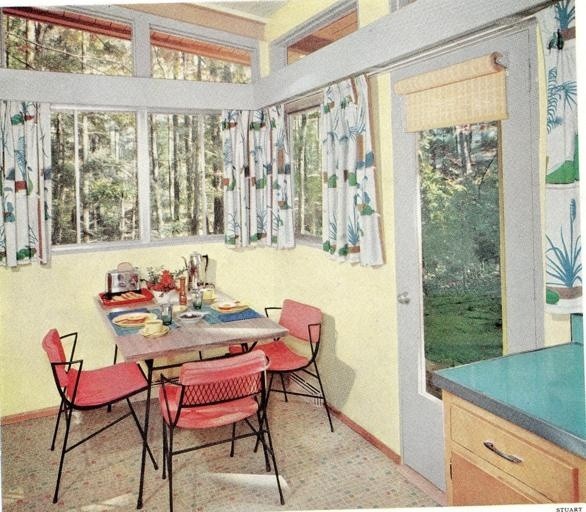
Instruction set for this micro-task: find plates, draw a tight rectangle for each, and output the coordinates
[112,312,158,327]
[139,326,169,338]
[211,301,249,315]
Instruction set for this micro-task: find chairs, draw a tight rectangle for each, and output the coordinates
[41,299,337,511]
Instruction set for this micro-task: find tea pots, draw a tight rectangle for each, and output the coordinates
[181,250,209,289]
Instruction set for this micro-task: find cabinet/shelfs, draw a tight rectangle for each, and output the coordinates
[430,371,586,506]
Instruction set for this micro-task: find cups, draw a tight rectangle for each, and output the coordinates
[144,320,163,334]
[203,285,216,302]
[193,293,202,309]
[161,304,172,324]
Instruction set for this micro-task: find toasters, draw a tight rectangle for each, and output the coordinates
[107,271,140,299]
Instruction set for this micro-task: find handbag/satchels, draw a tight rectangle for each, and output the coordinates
[108,262,140,294]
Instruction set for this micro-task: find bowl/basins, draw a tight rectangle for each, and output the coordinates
[177,312,203,323]
